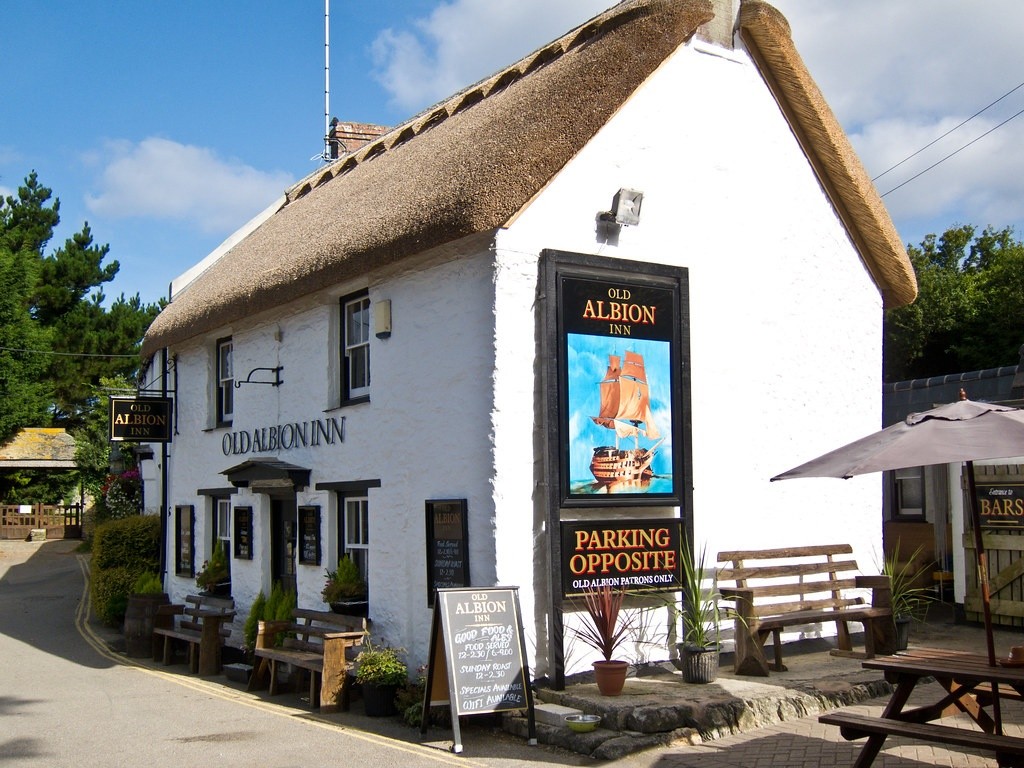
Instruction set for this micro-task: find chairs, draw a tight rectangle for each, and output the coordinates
[150,595,238,677]
[244,607,372,716]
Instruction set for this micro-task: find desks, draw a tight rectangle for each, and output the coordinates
[849,649,1024,768]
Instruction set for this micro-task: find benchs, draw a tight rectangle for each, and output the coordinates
[715,543,891,671]
[819,683,1024,768]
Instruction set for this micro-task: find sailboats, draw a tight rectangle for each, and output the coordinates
[589,350,667,484]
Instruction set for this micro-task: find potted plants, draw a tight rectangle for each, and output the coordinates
[320,554,369,617]
[123,567,175,660]
[865,534,955,652]
[550,523,763,696]
[338,638,410,717]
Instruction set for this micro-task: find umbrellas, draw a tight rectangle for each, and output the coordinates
[768,389,1024,736]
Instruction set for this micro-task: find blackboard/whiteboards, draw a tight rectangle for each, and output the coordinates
[424,586,534,716]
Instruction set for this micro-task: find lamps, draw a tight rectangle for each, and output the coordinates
[599,191,646,230]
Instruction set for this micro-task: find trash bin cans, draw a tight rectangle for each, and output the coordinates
[123,593,175,658]
